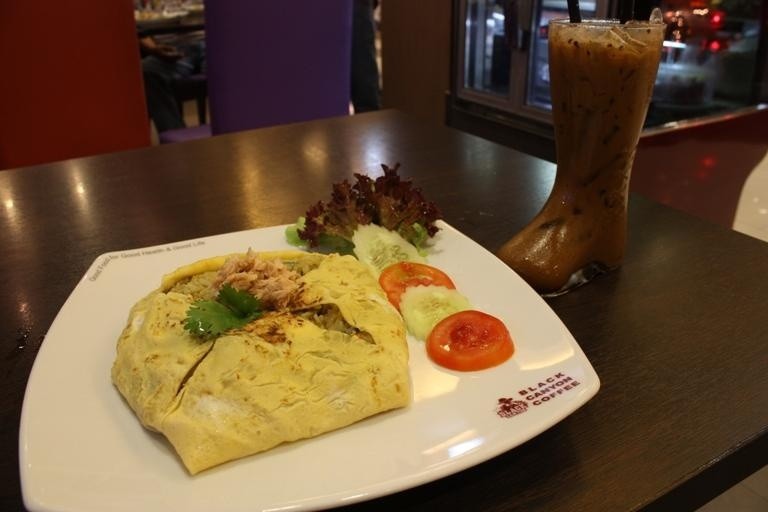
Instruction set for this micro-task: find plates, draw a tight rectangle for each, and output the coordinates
[19,218,601,510]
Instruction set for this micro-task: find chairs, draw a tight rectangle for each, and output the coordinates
[203,0,355,137]
[732,153,768,244]
[0,0,153,171]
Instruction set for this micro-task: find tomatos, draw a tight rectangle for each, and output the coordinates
[379,261,454,315]
[424,311,515,372]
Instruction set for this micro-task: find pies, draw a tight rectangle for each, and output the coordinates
[113,251,409,476]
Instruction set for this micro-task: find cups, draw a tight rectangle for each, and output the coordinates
[498,17,667,303]
